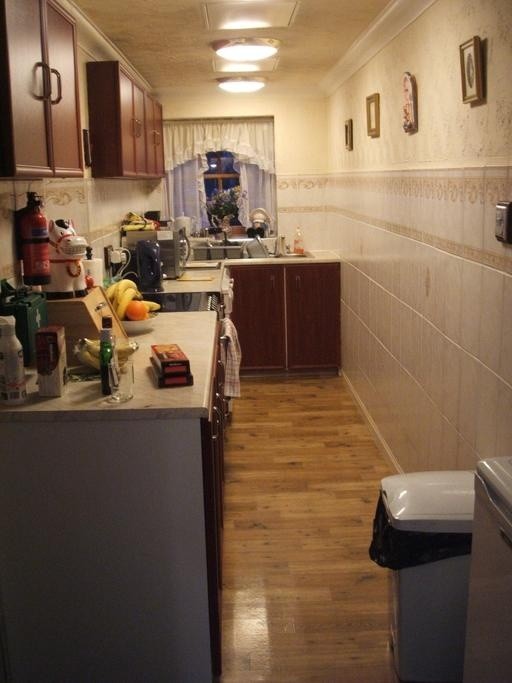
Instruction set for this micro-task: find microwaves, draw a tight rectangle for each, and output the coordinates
[124,224,191,279]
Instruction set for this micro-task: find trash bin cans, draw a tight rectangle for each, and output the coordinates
[378,470,476,683]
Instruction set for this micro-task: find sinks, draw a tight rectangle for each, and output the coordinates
[272,254,308,258]
[191,237,251,259]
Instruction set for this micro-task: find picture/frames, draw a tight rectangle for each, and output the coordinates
[458,33,486,105]
[343,118,353,150]
[365,92,381,138]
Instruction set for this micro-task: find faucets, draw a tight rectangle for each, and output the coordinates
[281,234,287,252]
[273,237,279,255]
[203,227,210,238]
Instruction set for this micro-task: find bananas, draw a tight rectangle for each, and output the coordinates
[105,279,143,321]
[74,337,139,374]
[145,300,161,312]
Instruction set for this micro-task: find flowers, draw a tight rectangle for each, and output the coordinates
[203,182,250,217]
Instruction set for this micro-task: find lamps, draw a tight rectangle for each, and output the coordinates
[211,36,282,63]
[214,75,269,94]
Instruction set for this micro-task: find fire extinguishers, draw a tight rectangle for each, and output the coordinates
[18,191,51,286]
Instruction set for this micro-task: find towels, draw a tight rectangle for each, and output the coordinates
[221,317,243,416]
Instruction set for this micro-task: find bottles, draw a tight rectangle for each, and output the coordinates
[204,227,209,237]
[200,229,204,236]
[285,243,290,253]
[0,322,27,408]
[96,313,120,395]
[293,226,304,254]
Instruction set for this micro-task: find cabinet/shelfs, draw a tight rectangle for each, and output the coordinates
[86,60,147,180]
[146,89,170,179]
[228,262,342,378]
[1,2,85,182]
[2,312,226,682]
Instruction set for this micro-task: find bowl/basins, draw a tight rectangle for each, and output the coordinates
[121,311,156,333]
